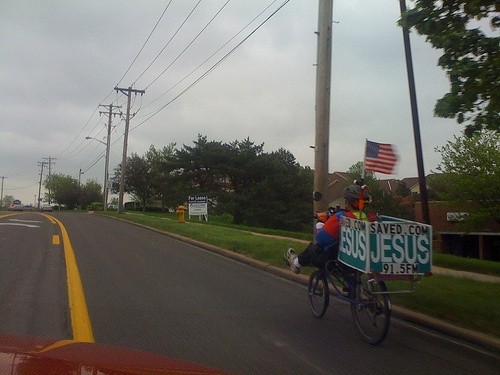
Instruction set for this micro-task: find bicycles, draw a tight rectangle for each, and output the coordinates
[307,190,392,347]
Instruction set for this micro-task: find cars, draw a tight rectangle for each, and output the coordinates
[40,204,52,212]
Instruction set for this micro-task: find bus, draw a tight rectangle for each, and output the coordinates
[13,200,21,210]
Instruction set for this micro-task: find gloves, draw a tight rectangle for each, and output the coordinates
[316,221,325,230]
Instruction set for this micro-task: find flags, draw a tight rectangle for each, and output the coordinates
[364,140,397,174]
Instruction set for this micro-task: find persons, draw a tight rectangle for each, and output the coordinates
[286,185,377,275]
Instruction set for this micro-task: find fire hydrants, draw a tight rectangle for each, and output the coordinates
[176,205,187,223]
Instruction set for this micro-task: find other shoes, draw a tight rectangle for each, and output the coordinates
[286,247,302,274]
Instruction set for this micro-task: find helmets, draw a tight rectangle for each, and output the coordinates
[343,185,369,203]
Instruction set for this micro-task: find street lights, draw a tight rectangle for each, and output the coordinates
[85,136,108,210]
